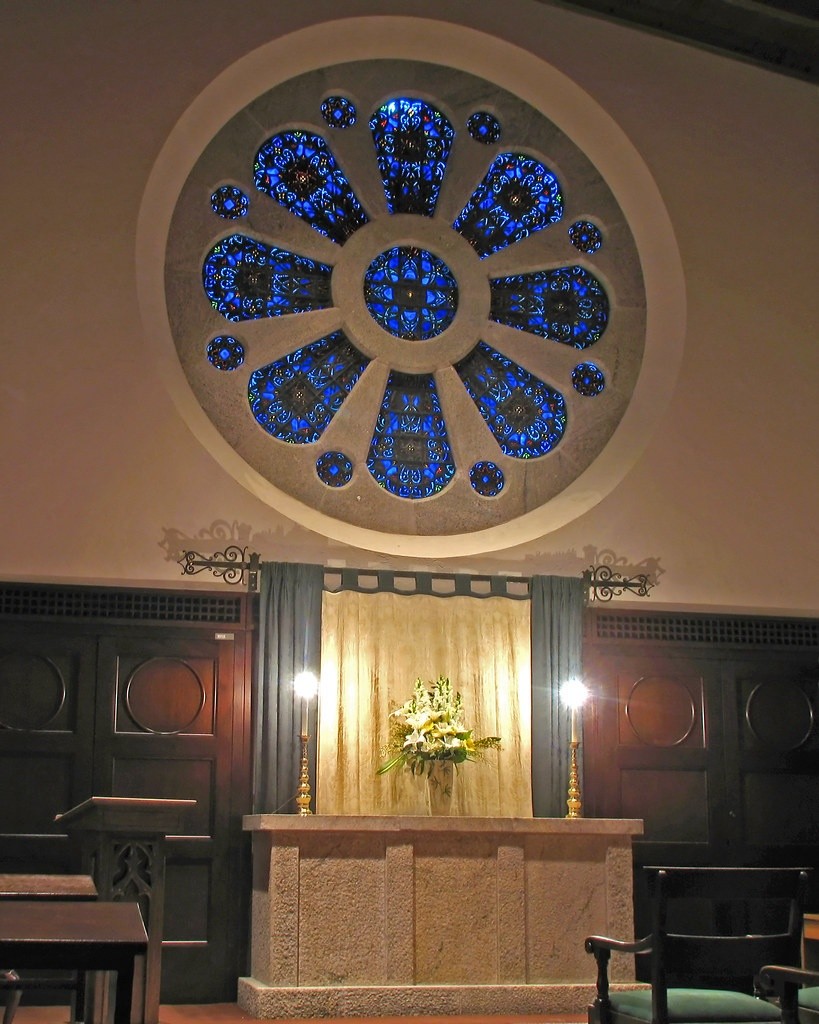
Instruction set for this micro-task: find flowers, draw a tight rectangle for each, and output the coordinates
[373,677,505,797]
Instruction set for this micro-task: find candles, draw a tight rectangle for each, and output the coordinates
[292,671,320,736]
[558,680,590,742]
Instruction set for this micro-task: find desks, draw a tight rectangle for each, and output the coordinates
[799,912,819,989]
[0,872,148,1024]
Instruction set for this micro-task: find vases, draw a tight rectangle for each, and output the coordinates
[423,760,454,816]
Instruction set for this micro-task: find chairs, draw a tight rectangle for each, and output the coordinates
[584,866,819,1024]
